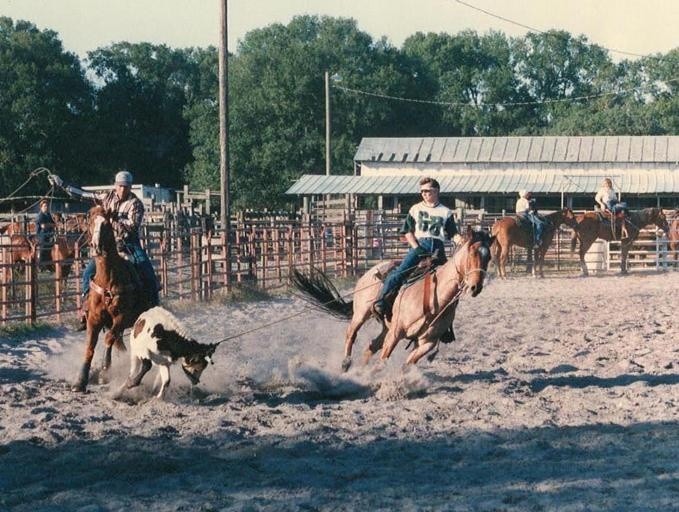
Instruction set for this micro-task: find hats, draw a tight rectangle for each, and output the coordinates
[115,171,133,187]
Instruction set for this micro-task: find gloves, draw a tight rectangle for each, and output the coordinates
[415,246,426,259]
[48,174,63,186]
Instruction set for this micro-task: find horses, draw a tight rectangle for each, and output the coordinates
[0,213,82,292]
[490,203,575,281]
[72,206,157,393]
[289,224,500,378]
[569,206,670,277]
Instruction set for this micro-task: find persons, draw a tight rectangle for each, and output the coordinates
[81,171,161,307]
[34,199,64,274]
[370,174,469,343]
[594,179,617,210]
[515,190,546,250]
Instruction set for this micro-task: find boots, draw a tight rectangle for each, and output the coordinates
[74,316,86,332]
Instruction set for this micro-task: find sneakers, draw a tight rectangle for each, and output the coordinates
[374,301,382,315]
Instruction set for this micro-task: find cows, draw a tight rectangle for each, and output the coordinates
[116,304,221,399]
